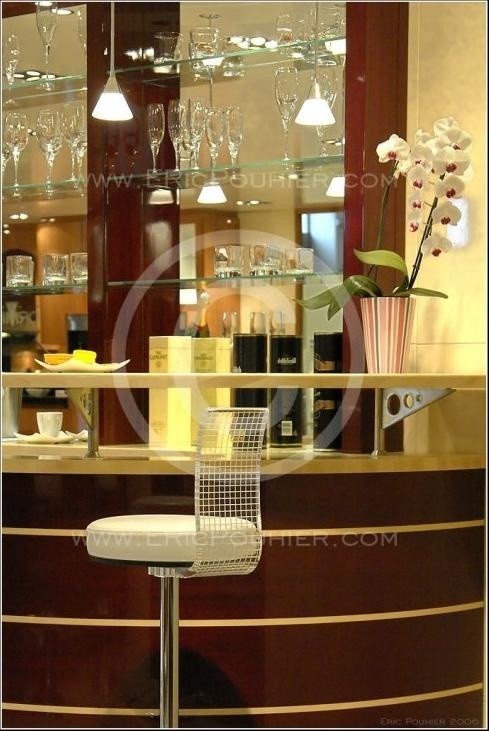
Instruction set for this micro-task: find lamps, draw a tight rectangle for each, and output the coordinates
[196,77,227,204]
[295,2,335,126]
[91,2,133,121]
[325,69,345,198]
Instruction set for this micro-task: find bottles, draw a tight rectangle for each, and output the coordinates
[196,306,210,338]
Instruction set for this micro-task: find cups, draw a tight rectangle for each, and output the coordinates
[221,311,287,337]
[271,1,348,47]
[211,242,315,278]
[152,23,217,73]
[3,252,89,291]
[35,410,64,438]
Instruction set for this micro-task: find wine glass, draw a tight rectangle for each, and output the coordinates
[2,1,87,108]
[144,95,246,181]
[0,102,90,201]
[271,60,344,181]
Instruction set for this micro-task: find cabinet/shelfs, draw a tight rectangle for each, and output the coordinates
[110,1,408,445]
[0,0,178,447]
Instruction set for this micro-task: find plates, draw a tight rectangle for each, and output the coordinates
[11,431,75,444]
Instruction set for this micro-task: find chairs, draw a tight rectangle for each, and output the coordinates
[87,406,270,729]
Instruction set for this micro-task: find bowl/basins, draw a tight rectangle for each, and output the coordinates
[44,349,98,365]
[34,357,132,372]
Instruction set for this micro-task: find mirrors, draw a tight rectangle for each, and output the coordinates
[2,3,87,400]
[170,1,346,449]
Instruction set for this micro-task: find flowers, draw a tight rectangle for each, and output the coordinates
[290,116,472,321]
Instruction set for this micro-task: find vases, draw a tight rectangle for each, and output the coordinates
[358,296,417,375]
